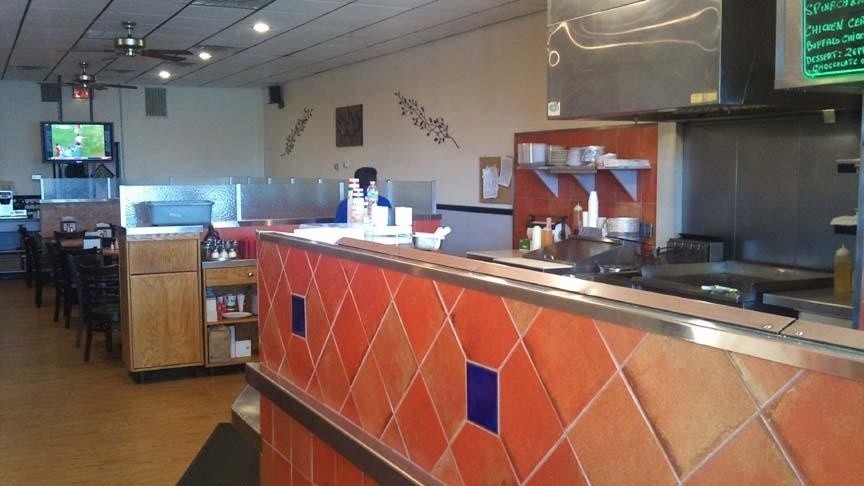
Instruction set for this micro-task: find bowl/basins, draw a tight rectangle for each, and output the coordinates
[410,232,445,251]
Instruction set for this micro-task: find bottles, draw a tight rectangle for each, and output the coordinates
[573,202,584,227]
[364,180,378,216]
[834,243,851,291]
[532,226,541,251]
[201,225,256,260]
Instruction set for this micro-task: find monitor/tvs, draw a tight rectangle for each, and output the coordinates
[40,121,114,164]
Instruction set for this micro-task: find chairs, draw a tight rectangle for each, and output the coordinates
[18,228,120,364]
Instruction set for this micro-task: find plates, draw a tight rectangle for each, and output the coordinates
[547,149,567,166]
[224,312,253,320]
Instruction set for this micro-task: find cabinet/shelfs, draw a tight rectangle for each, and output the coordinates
[120,237,258,382]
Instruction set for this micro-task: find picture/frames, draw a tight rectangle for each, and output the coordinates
[334,104,361,148]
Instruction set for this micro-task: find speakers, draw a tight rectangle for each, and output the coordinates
[268,86,280,103]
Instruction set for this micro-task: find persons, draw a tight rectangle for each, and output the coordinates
[55,124,87,157]
[335,167,392,225]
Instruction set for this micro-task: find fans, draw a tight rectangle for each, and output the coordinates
[34,19,191,90]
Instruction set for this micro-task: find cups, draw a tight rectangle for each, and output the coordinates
[583,190,608,234]
[740,280,760,301]
[346,178,363,224]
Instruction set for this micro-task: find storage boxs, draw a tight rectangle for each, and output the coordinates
[0,217,41,280]
